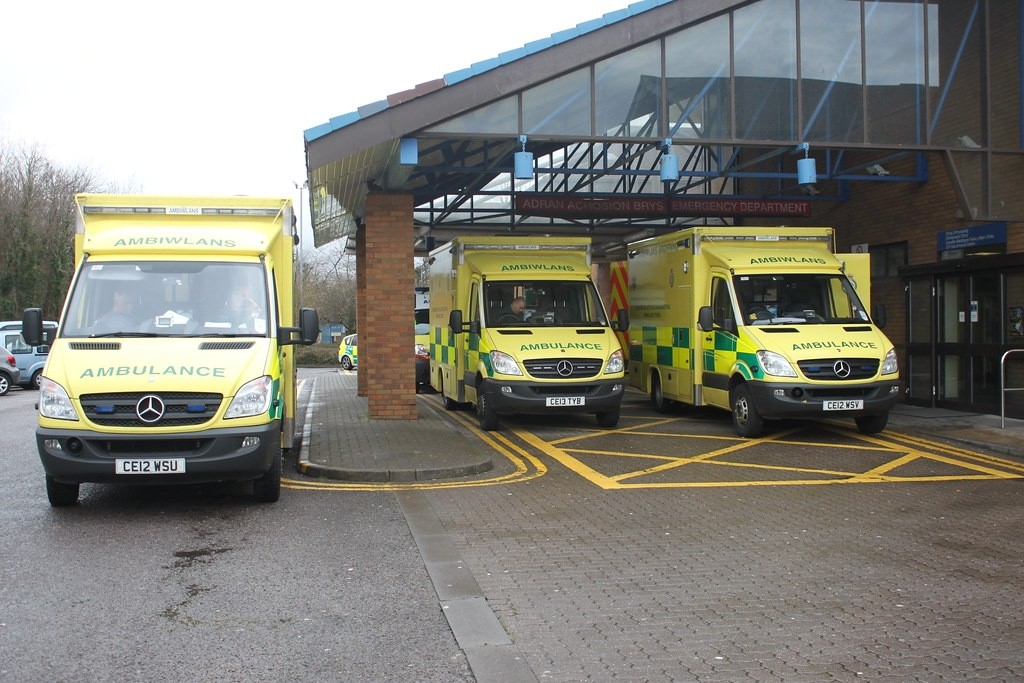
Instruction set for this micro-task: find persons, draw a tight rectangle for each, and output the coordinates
[493,297,527,323]
[111,286,136,317]
[225,277,262,322]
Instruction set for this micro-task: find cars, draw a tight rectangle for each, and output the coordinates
[338,333,358,370]
[0,344,21,396]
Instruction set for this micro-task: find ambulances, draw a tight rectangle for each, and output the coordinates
[19,191,321,508]
[425,235,629,430]
[606,226,903,438]
[415,286,431,394]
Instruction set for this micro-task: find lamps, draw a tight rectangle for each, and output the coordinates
[791,142,817,185]
[514,135,533,178]
[415,236,426,249]
[656,139,679,182]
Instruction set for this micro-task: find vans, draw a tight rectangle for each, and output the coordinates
[0,320,59,391]
